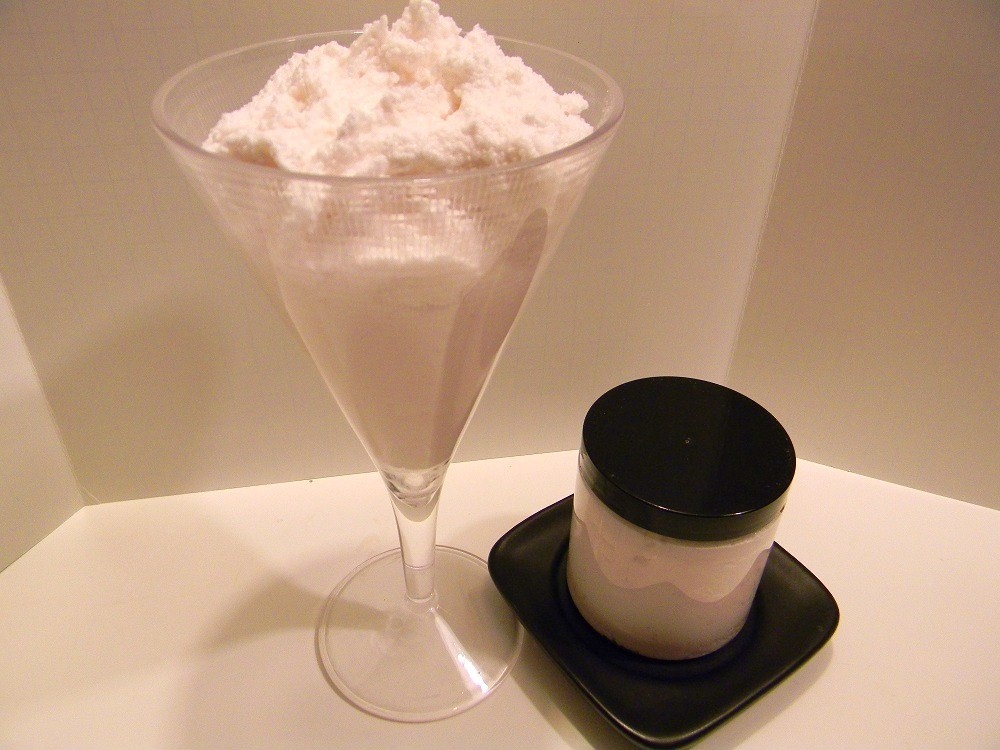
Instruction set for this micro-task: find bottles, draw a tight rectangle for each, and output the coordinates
[563,374,797,663]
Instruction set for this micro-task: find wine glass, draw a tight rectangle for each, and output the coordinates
[150,31,627,725]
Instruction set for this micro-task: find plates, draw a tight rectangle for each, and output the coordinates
[486,493,840,750]
